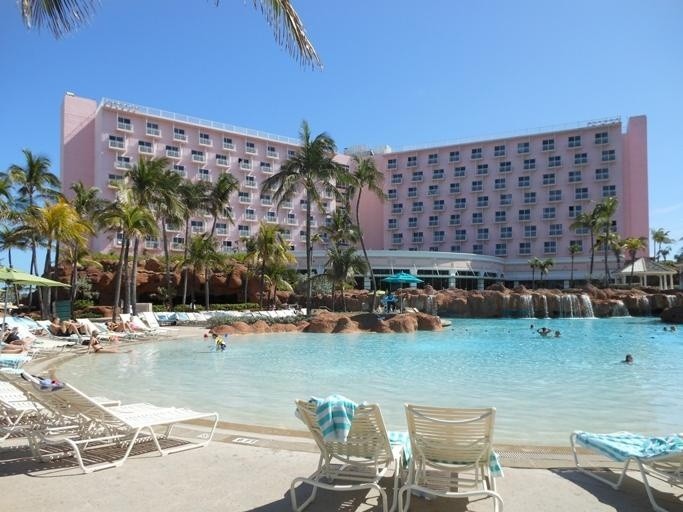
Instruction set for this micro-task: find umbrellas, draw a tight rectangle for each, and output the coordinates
[382,270,424,314]
[0,264,72,327]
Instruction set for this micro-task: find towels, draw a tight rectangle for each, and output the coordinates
[576,432,683,462]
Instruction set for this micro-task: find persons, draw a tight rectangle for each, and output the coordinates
[49,316,82,338]
[89,330,132,353]
[74,319,88,335]
[386,291,396,313]
[212,333,226,354]
[536,327,552,336]
[556,330,562,338]
[622,354,634,364]
[106,321,134,333]
[2,323,25,345]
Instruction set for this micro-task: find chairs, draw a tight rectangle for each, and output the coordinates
[0,293,182,368]
[155,308,306,328]
[570,430,683,512]
[1,367,221,475]
[289,396,406,511]
[398,401,504,511]
